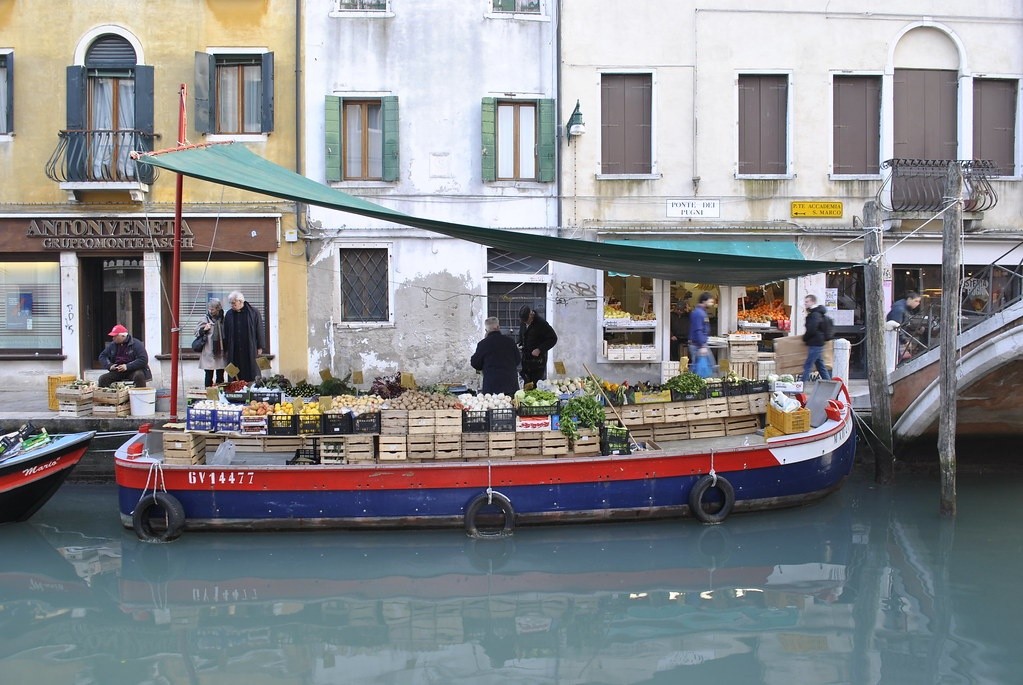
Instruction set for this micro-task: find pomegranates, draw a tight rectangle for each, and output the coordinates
[242,400,273,430]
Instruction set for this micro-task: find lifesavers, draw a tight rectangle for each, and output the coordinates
[464,492,516,535]
[132,491,186,541]
[687,474,737,524]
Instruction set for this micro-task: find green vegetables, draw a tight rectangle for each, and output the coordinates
[658,372,707,394]
[515,388,607,441]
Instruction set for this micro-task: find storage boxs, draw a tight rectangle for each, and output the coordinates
[705,334,776,378]
[607,349,659,361]
[152,380,810,464]
[47,376,131,418]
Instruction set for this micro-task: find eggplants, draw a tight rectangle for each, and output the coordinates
[636,381,666,391]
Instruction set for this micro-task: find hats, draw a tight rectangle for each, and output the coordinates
[518,305,531,324]
[108,324,129,336]
[677,302,686,310]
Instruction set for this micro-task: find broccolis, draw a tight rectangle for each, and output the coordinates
[766,373,793,382]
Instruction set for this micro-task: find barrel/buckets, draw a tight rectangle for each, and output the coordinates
[128,387,157,417]
[155,389,171,412]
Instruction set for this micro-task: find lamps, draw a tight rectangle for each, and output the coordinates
[566,99,586,146]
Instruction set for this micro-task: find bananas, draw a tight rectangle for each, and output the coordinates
[694,284,714,291]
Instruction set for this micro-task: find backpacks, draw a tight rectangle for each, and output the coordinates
[811,310,836,341]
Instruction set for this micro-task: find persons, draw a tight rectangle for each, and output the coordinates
[98,324,152,388]
[471,316,521,400]
[517,304,558,390]
[886,289,922,367]
[802,295,831,382]
[670,301,692,363]
[193,298,225,388]
[222,291,265,382]
[688,291,716,379]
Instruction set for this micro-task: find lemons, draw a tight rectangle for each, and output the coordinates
[275,401,322,434]
[605,306,630,318]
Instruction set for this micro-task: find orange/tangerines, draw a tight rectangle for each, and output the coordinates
[738,298,788,320]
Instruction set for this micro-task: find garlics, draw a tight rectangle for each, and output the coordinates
[189,400,243,431]
[458,392,513,421]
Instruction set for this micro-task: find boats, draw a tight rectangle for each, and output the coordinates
[114,380,858,541]
[122,522,851,608]
[0,430,96,525]
[0,525,95,604]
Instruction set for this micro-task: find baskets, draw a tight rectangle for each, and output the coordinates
[671,379,770,401]
[48,375,75,410]
[519,400,562,416]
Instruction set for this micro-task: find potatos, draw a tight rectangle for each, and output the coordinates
[328,390,461,414]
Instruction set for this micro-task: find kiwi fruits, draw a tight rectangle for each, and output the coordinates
[748,317,771,323]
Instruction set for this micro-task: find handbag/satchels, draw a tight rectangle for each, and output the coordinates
[693,353,713,379]
[192,316,209,353]
